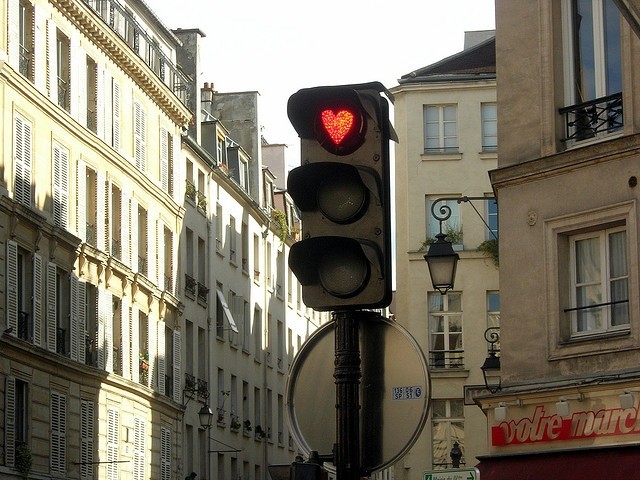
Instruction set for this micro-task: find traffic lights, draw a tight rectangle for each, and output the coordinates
[286,80,394,310]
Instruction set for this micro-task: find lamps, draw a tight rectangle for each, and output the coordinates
[180,389,217,429]
[422,196,497,293]
[479,326,500,391]
